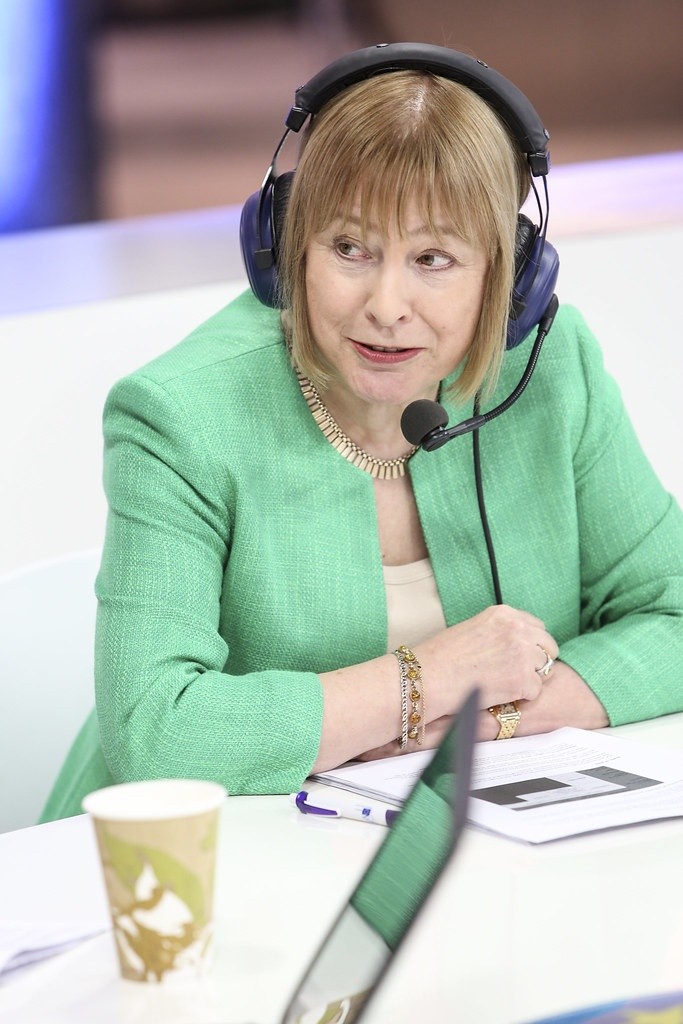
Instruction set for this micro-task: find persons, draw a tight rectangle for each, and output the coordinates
[40,69,683,826]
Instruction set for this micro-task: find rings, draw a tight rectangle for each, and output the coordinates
[537,649,554,674]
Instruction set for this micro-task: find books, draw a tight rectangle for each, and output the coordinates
[314,735,683,842]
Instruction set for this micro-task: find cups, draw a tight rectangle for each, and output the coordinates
[83,778,229,982]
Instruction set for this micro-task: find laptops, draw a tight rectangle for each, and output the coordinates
[281,686,488,1024]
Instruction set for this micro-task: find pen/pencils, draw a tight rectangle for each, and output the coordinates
[296,790,402,827]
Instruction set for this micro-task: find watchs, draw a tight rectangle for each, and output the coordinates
[487,702,521,740]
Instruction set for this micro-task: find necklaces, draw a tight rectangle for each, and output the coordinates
[293,365,421,480]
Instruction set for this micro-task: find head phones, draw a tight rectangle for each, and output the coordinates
[240,41,560,354]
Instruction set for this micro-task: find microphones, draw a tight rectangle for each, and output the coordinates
[400,332,545,451]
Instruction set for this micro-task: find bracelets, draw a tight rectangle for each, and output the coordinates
[392,647,426,752]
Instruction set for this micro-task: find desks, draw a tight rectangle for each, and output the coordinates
[0,705,683,1024]
